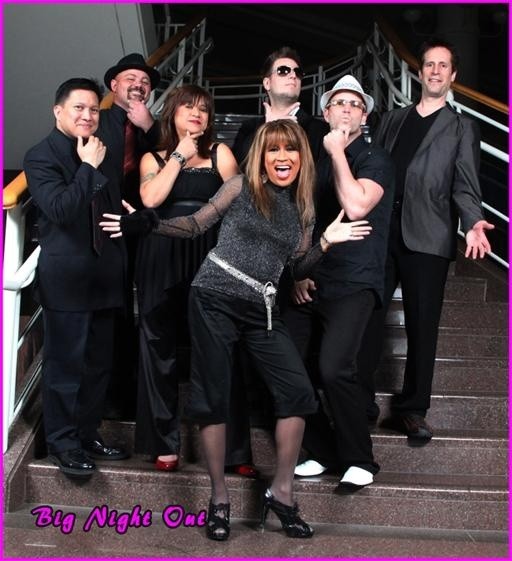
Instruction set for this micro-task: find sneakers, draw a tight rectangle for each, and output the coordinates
[293,459,331,477]
[396,414,432,439]
[339,466,375,486]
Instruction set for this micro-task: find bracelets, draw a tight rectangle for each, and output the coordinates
[320,231,333,246]
[169,150,187,167]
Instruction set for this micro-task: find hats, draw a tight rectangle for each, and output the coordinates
[320,74,375,115]
[104,54,160,91]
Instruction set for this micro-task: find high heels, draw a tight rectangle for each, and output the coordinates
[207,499,230,540]
[260,488,314,538]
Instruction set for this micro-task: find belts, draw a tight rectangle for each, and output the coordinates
[209,253,276,298]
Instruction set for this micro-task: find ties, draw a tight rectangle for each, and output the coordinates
[123,120,136,177]
[91,197,106,257]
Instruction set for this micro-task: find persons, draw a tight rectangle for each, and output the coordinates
[369,37,497,441]
[290,73,399,488]
[136,82,259,479]
[22,74,132,478]
[234,41,332,167]
[99,119,374,540]
[96,50,162,423]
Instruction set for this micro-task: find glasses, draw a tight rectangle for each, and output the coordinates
[325,99,366,112]
[266,65,304,80]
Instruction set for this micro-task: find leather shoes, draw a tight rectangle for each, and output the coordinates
[156,455,179,469]
[234,465,260,477]
[79,438,127,460]
[46,449,96,476]
